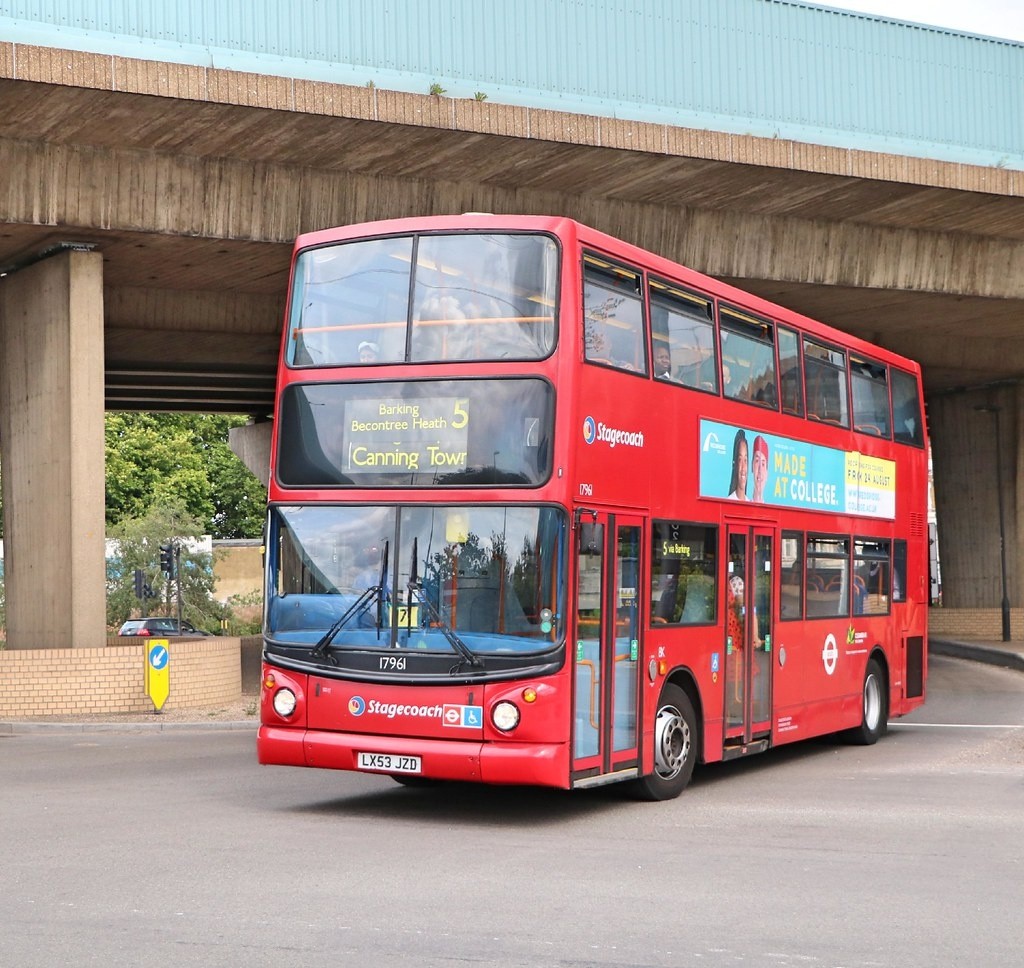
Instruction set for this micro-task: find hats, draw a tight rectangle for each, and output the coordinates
[753,436,768,460]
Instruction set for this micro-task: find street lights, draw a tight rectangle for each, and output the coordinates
[973,402,1011,642]
[493,449,500,469]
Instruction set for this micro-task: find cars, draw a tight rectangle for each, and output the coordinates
[119,617,215,637]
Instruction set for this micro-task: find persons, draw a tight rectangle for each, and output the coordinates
[585,327,634,370]
[653,346,684,385]
[727,572,761,717]
[722,364,731,388]
[789,560,801,585]
[751,436,769,503]
[357,341,379,363]
[727,429,751,501]
[857,545,900,602]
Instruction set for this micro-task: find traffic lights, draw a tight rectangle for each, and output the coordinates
[132,570,142,591]
[159,544,173,572]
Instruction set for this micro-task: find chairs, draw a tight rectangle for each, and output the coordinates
[825,582,860,616]
[753,400,881,435]
[830,574,865,591]
[794,581,819,593]
[799,574,824,591]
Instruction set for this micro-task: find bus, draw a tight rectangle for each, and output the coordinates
[256,212,930,802]
